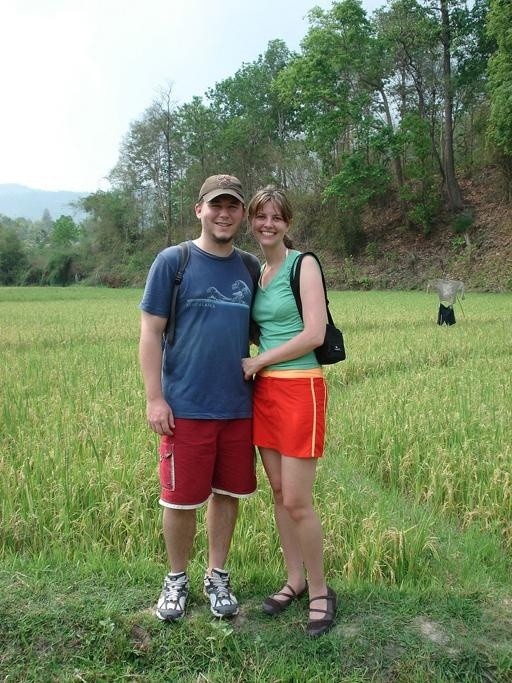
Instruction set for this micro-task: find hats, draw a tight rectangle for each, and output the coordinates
[198,175,246,205]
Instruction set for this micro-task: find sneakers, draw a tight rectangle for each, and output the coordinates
[203,567,239,617]
[155,571,189,621]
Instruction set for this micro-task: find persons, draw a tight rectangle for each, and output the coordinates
[425,270,466,326]
[236,182,337,638]
[136,171,261,621]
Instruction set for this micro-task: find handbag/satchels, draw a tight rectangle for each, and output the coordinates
[290,252,347,365]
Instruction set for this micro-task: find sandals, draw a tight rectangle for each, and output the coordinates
[262,578,337,637]
